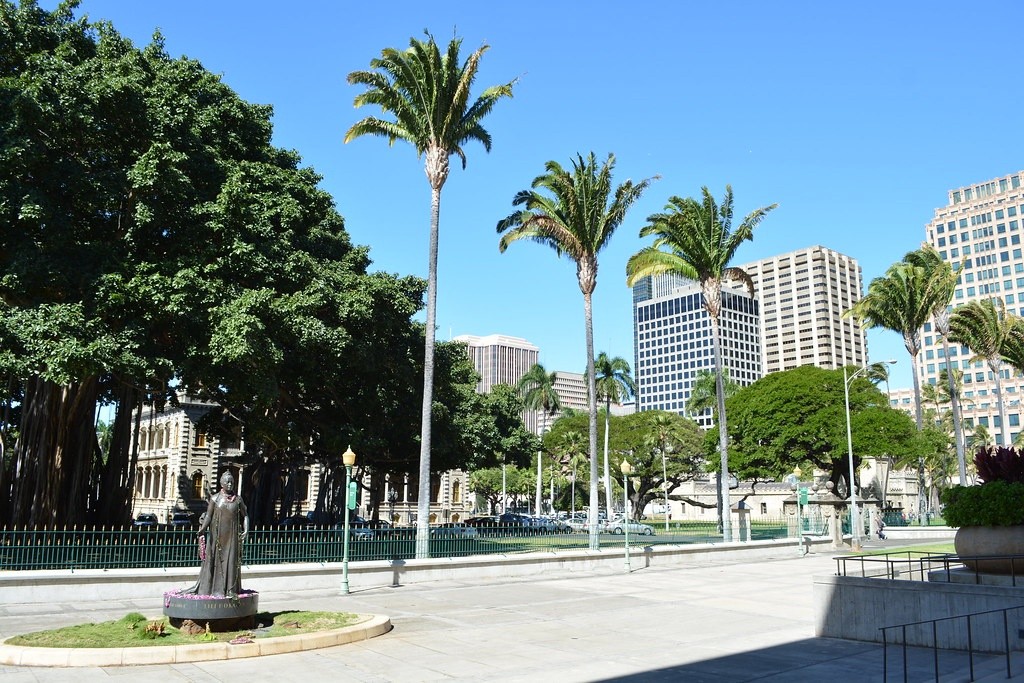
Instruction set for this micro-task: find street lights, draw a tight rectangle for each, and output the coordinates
[793,463,804,556]
[619,458,631,572]
[842,359,897,551]
[340,445,357,595]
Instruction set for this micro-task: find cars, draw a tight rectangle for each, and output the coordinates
[135,506,656,535]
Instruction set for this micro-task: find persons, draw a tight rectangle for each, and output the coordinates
[175,468,250,596]
